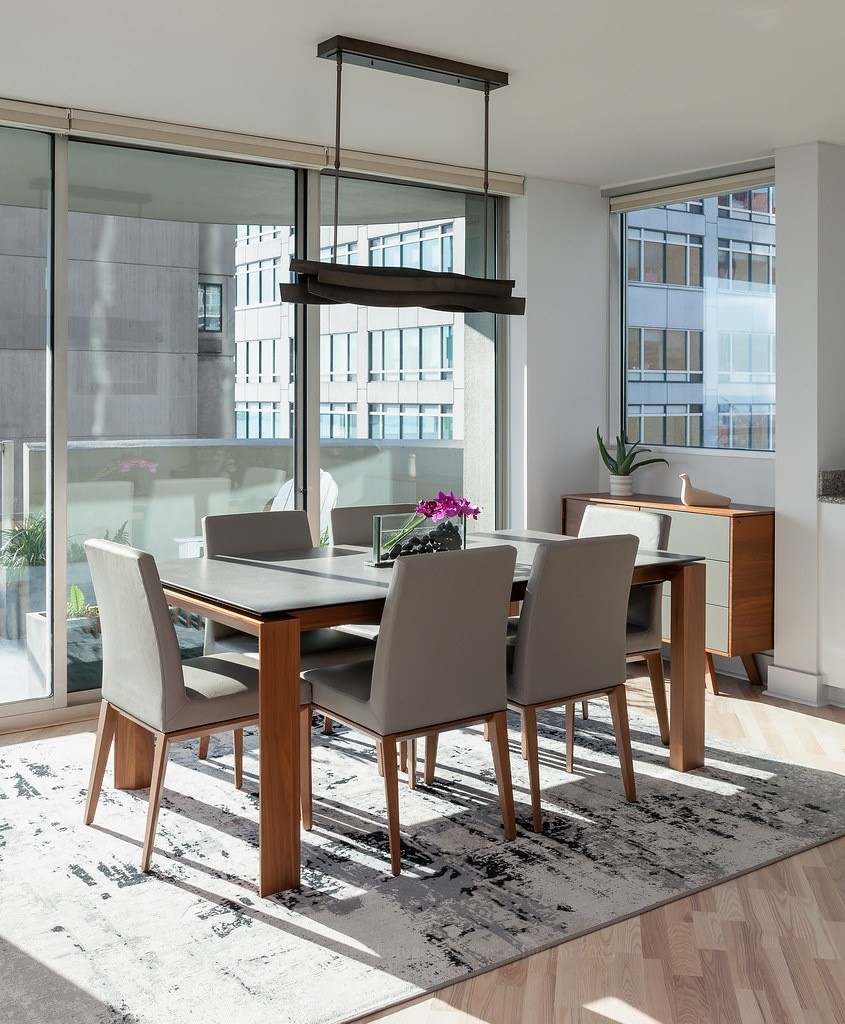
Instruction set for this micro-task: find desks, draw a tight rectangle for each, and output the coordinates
[113,529,706,898]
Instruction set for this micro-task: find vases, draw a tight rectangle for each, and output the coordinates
[364,512,466,568]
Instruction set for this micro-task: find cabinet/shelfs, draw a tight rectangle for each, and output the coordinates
[560,492,775,695]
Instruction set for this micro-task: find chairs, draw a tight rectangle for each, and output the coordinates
[328,504,427,772]
[175,467,339,558]
[484,502,672,759]
[508,533,639,833]
[301,544,517,877]
[198,510,418,791]
[83,539,312,873]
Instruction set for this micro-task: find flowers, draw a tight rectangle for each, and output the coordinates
[382,491,482,552]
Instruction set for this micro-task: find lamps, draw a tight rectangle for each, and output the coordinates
[279,34,526,315]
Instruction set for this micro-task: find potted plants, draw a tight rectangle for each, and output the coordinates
[27,584,204,692]
[596,426,669,496]
[0,506,131,640]
